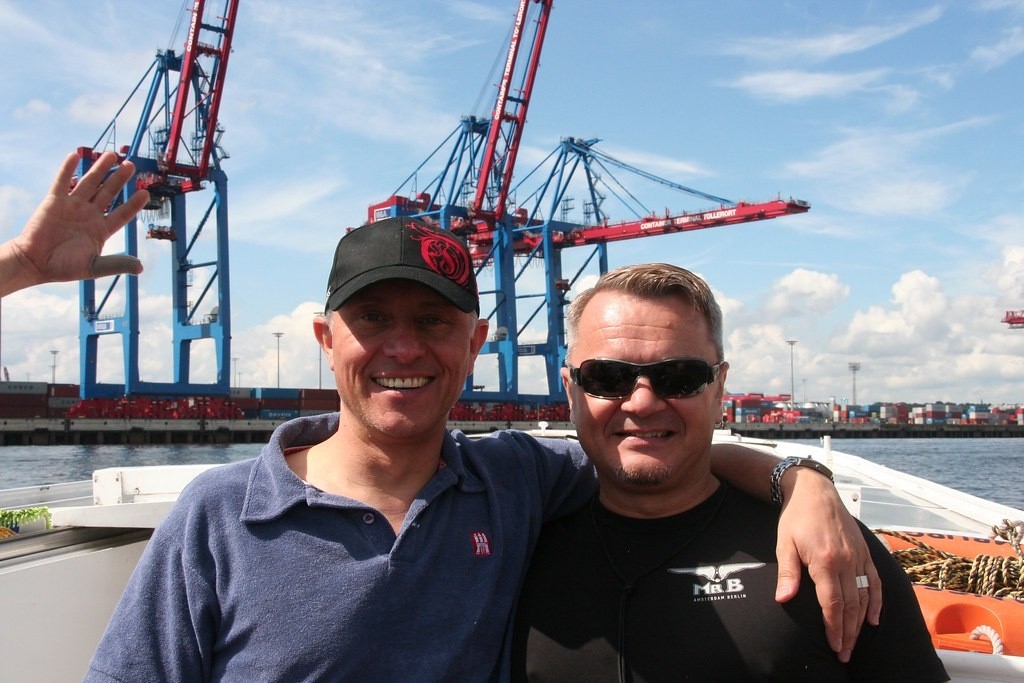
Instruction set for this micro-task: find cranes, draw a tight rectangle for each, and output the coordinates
[366,1,811,424]
[65,0,250,420]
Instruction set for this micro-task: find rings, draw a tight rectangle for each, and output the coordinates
[854,574,872,590]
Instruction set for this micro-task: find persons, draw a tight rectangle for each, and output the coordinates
[83,218,882,683]
[1,150,152,297]
[508,260,949,682]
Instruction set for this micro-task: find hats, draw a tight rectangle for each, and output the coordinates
[324,215,480,322]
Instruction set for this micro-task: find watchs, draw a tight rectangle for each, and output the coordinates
[770,454,836,506]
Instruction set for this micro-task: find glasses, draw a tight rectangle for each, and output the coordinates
[567,358,721,400]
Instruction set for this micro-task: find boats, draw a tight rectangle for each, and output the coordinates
[0,421,1023,683]
